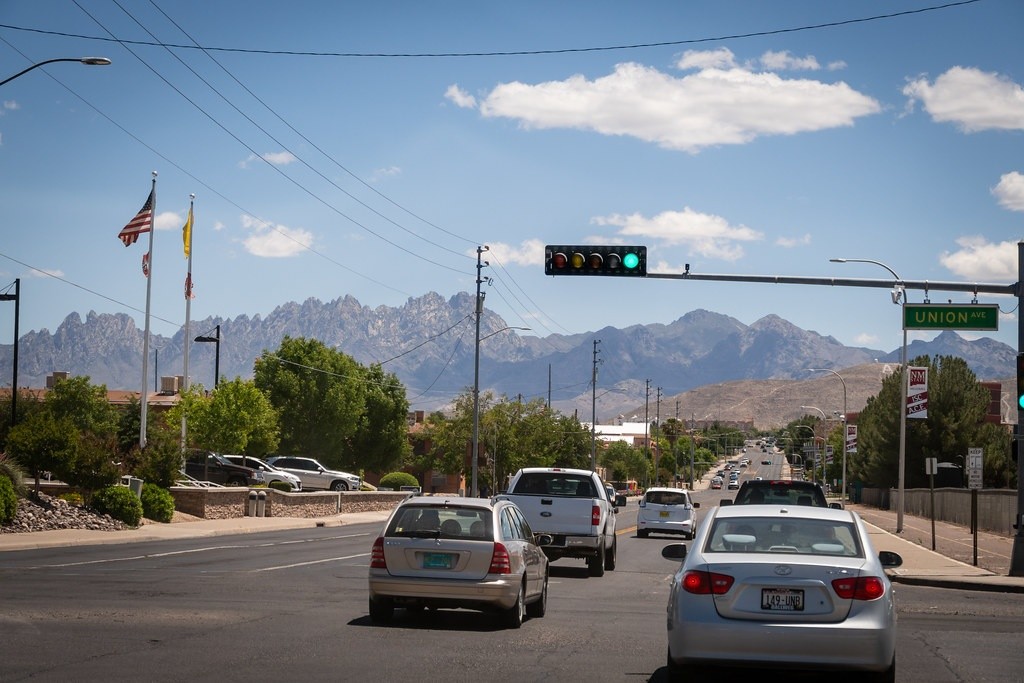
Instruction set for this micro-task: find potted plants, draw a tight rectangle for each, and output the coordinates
[379,471,422,496]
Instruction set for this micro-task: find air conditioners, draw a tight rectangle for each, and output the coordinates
[46,372,71,390]
[161,375,190,394]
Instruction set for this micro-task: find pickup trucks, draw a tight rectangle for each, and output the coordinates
[495,467,627,578]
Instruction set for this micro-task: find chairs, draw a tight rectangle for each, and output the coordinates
[575,481,592,495]
[812,544,847,554]
[440,520,462,536]
[470,520,486,537]
[721,533,758,552]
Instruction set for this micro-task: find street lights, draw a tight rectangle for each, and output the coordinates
[801,369,846,510]
[829,256,907,535]
[471,326,532,498]
[0,278,22,430]
[591,387,628,472]
[799,405,826,496]
[794,425,816,484]
[194,324,220,391]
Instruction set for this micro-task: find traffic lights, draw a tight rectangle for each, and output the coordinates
[544,245,648,276]
[1016,354,1024,411]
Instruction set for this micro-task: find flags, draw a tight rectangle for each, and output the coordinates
[117,190,153,247]
[182,209,195,259]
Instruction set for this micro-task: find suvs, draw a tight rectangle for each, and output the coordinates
[720,479,842,546]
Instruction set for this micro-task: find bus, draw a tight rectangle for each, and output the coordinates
[608,481,637,491]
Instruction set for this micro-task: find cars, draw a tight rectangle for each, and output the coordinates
[604,486,620,503]
[637,486,700,540]
[662,504,904,683]
[207,454,302,492]
[185,448,266,487]
[711,436,774,490]
[263,455,361,492]
[367,491,552,630]
